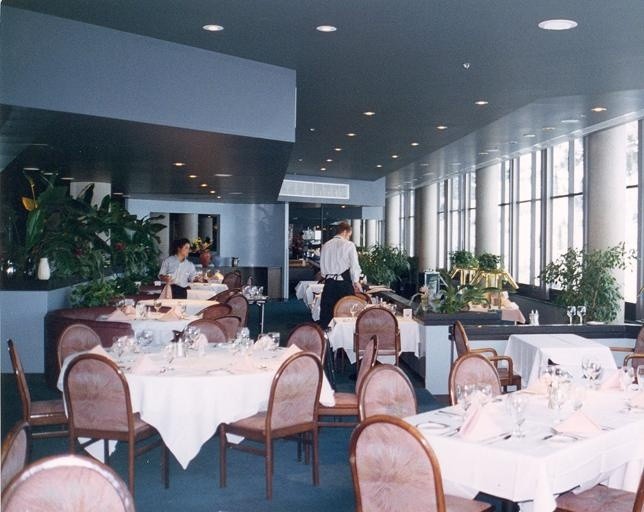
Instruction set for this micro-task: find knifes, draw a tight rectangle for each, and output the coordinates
[488,435,511,447]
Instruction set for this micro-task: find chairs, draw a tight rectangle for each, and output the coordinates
[63,353,170,496]
[172,319,229,344]
[609,326,644,371]
[454,320,522,391]
[358,364,417,421]
[0,422,29,489]
[448,352,501,407]
[179,320,227,345]
[226,294,249,327]
[207,289,238,304]
[1,455,136,512]
[283,322,327,461]
[222,269,243,290]
[333,296,368,320]
[56,322,102,369]
[219,351,324,500]
[553,466,644,511]
[349,414,493,512]
[304,335,379,466]
[215,316,241,339]
[6,338,68,465]
[193,303,233,320]
[354,305,402,374]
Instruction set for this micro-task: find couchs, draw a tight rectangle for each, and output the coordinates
[107,293,158,310]
[136,286,165,300]
[43,307,135,395]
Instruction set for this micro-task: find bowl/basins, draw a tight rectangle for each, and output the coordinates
[211,256,222,267]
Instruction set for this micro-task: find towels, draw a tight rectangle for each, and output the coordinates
[124,304,138,316]
[159,284,172,301]
[190,331,207,351]
[459,406,496,448]
[598,368,625,395]
[159,309,177,323]
[225,353,254,376]
[555,407,599,447]
[88,345,109,357]
[131,354,154,379]
[526,373,555,401]
[253,332,276,353]
[106,308,127,322]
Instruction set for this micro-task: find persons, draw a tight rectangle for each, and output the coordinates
[159,237,196,299]
[293,231,304,259]
[319,221,363,331]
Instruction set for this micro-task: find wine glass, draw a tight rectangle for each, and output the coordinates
[160,343,172,376]
[475,383,493,407]
[113,335,128,366]
[141,330,154,350]
[268,332,280,352]
[577,305,586,325]
[456,384,473,422]
[636,365,644,397]
[567,306,576,325]
[619,365,634,399]
[507,393,526,438]
[541,365,558,399]
[237,327,250,353]
[185,326,200,349]
[582,360,604,392]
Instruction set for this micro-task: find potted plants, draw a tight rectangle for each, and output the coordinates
[124,249,142,287]
[0,207,22,281]
[23,171,96,281]
[418,285,429,311]
[69,183,94,257]
[470,253,520,291]
[448,247,480,284]
[18,170,36,280]
[104,195,138,252]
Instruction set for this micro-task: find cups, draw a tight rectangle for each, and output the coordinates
[224,256,232,267]
[231,257,240,267]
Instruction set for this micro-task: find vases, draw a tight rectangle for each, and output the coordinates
[200,254,211,267]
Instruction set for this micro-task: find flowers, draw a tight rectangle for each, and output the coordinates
[190,236,213,253]
